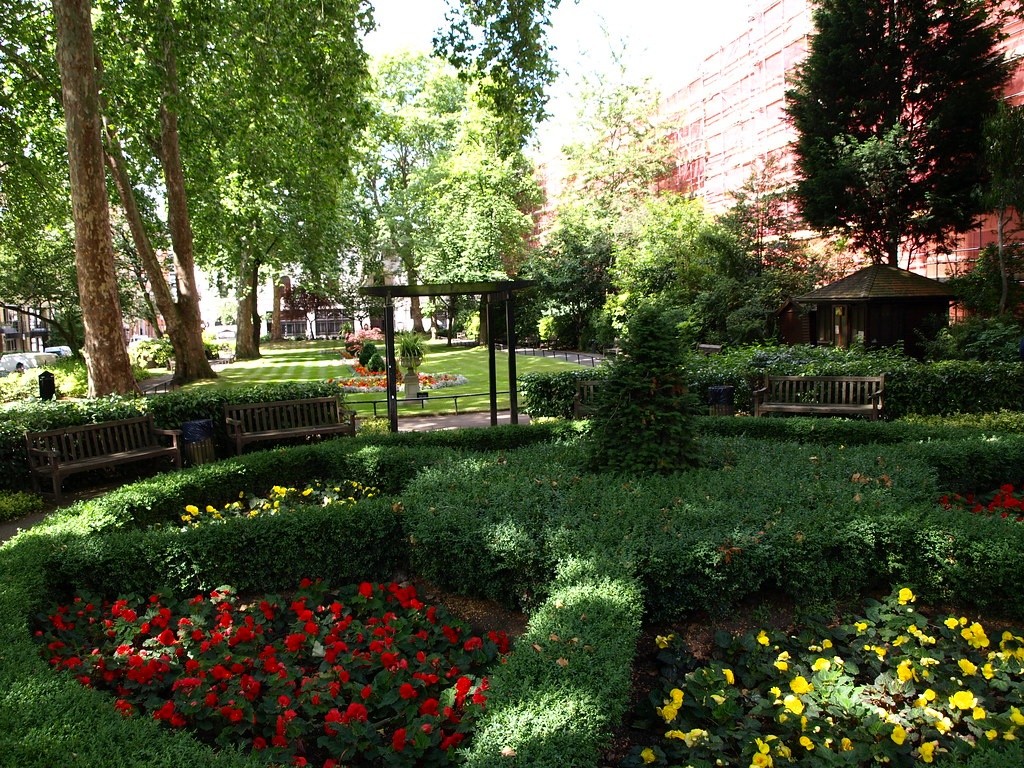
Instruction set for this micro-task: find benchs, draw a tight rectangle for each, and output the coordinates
[222,396,357,457]
[752,372,885,421]
[24,413,183,505]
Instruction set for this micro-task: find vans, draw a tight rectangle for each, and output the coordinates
[45,345,73,360]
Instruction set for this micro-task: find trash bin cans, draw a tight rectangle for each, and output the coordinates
[38,371,55,401]
[182,418,217,464]
[708,385,735,416]
